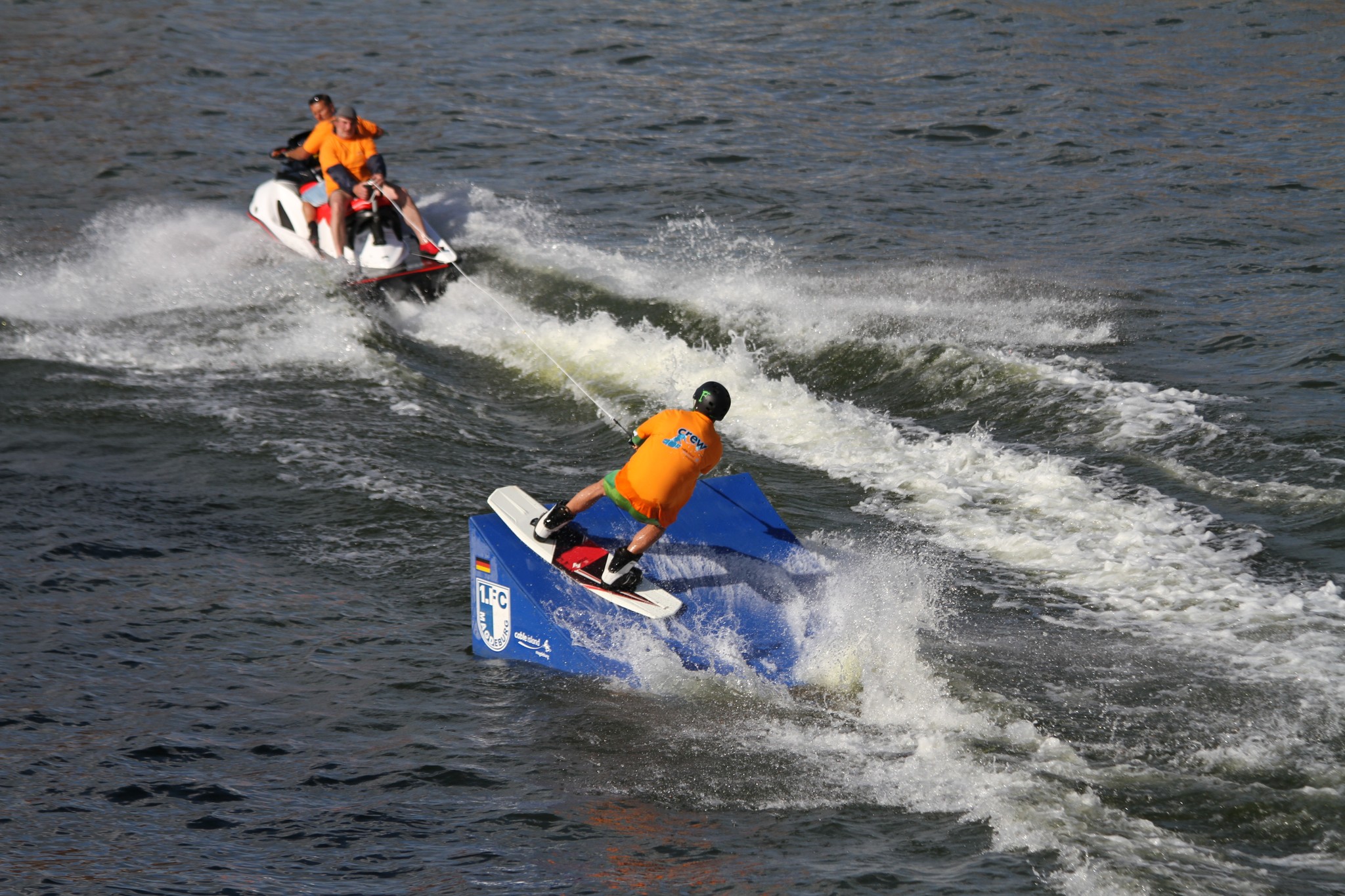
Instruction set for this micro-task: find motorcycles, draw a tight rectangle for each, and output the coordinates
[246,126,462,299]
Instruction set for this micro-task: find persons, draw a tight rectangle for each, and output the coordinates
[268,93,456,264]
[534,382,731,593]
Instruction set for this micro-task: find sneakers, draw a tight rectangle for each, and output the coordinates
[419,242,453,262]
[533,499,573,543]
[601,545,645,591]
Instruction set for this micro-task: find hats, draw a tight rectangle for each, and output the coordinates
[338,106,358,123]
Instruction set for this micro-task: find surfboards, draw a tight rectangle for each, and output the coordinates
[487,486,684,620]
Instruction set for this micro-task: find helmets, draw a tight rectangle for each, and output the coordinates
[693,381,732,421]
